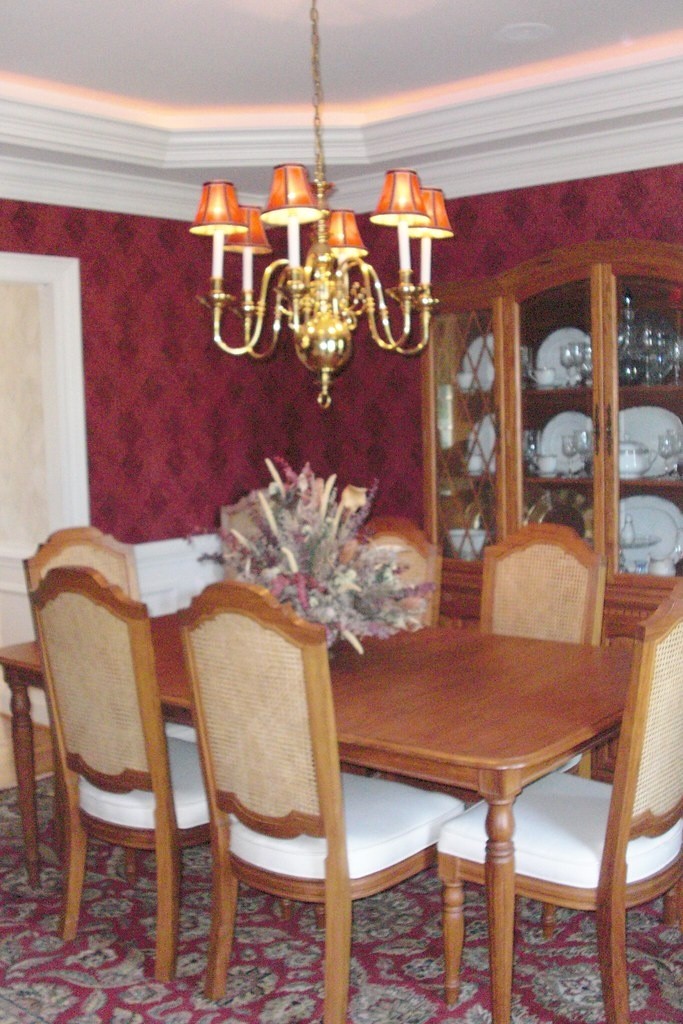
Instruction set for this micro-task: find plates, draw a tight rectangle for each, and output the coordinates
[536,327,591,389]
[463,333,496,392]
[538,411,595,474]
[468,413,497,474]
[618,406,683,478]
[619,495,683,575]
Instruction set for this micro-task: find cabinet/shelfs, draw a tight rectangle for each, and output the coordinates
[420,236,683,641]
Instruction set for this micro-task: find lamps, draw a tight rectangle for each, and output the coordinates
[188,0,454,411]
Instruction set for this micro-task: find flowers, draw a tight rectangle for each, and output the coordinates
[180,452,435,654]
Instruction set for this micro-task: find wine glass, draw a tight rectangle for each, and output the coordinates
[659,435,675,481]
[573,430,593,478]
[668,429,683,479]
[561,343,592,388]
[641,319,683,387]
[562,435,579,479]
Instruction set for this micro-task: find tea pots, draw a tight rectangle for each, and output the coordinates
[618,434,656,479]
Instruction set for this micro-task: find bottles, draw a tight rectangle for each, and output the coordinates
[618,297,643,386]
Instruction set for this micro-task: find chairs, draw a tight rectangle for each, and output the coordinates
[359,514,444,623]
[28,564,239,985]
[175,580,466,1024]
[436,583,683,1024]
[23,525,139,642]
[478,522,608,779]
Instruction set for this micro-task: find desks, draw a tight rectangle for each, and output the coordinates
[0,611,634,1024]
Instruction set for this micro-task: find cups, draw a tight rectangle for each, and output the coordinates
[448,529,487,560]
[465,455,482,475]
[538,455,557,477]
[529,368,556,390]
